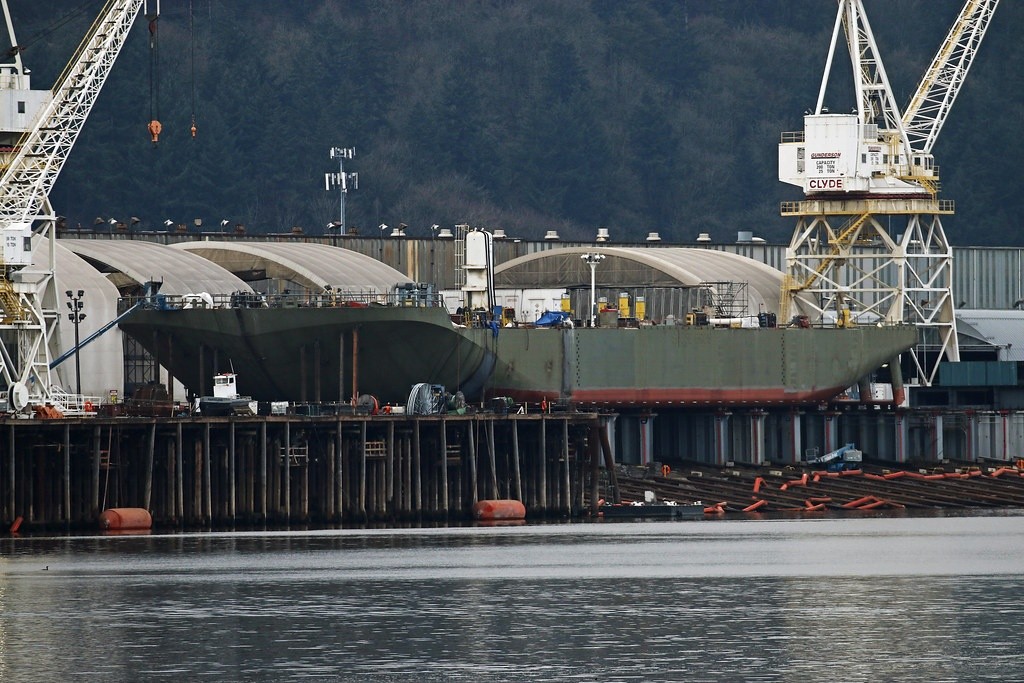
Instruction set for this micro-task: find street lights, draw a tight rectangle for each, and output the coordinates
[324,144,361,234]
[580,252,606,329]
[65,288,87,398]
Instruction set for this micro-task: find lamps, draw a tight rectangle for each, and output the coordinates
[379,223,388,236]
[431,224,439,237]
[851,107,858,114]
[108,218,117,232]
[220,220,229,232]
[164,220,173,230]
[93,217,105,230]
[821,107,828,114]
[922,299,928,307]
[57,215,67,228]
[959,301,965,308]
[130,217,140,230]
[1013,299,1023,310]
[327,221,342,235]
[398,223,407,236]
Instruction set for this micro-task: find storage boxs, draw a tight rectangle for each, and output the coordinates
[843,450,862,462]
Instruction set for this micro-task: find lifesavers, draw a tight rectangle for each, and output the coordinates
[542,401,547,411]
[662,465,670,475]
[84,401,92,412]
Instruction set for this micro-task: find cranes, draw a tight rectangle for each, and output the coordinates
[768,0,999,359]
[0,1,152,414]
